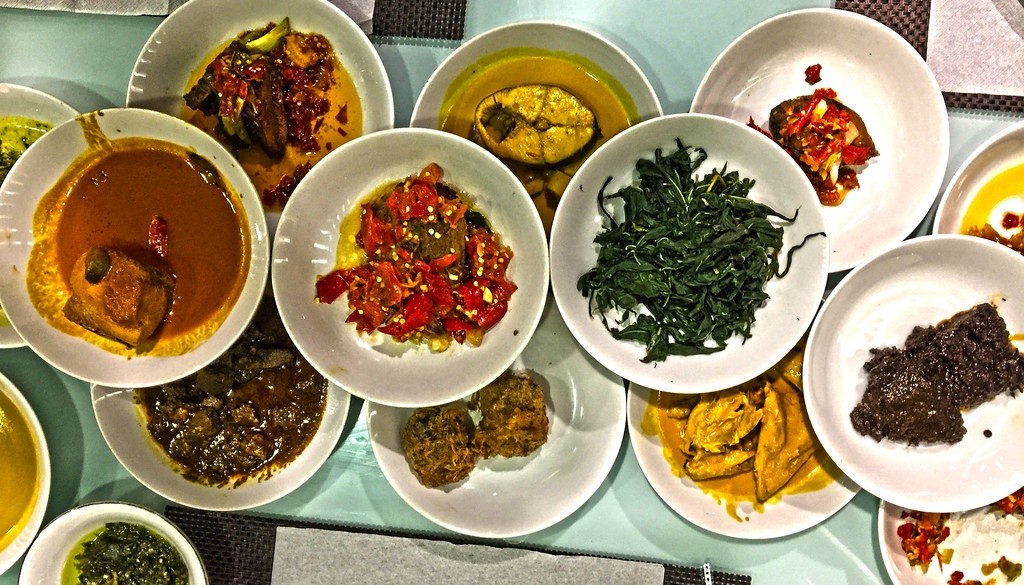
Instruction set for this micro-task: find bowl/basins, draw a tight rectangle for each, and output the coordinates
[878,489,1024,585]
[1,83,84,348]
[367,285,627,538]
[16,501,208,585]
[931,116,1024,256]
[272,127,549,408]
[125,2,395,246]
[691,8,951,273]
[92,282,353,513]
[802,235,1024,514]
[408,21,665,245]
[627,324,861,539]
[547,112,829,394]
[0,373,51,575]
[0,109,269,390]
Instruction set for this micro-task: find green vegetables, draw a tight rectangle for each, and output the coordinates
[576,137,828,363]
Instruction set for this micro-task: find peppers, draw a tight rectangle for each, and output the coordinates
[747,62,872,171]
[316,181,520,339]
